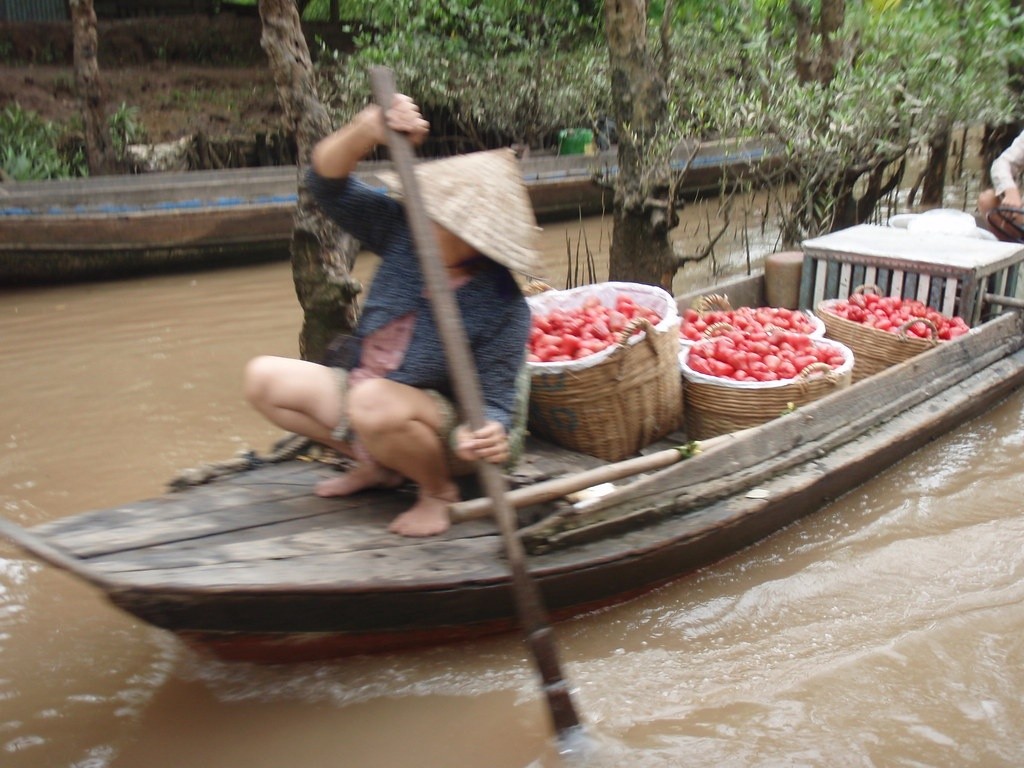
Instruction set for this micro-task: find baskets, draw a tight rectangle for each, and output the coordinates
[697,293,827,337]
[679,322,854,440]
[523,280,684,463]
[817,284,950,387]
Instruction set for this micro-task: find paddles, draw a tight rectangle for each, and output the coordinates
[445,431,743,524]
[368,62,581,739]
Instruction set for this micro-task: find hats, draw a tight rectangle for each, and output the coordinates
[377,147,547,280]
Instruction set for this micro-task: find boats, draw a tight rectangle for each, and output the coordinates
[0,206,1024,670]
[3,132,804,289]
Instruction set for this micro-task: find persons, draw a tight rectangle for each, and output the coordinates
[978,128,1024,241]
[244,95,548,536]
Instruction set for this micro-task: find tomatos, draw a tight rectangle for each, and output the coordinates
[681,306,847,384]
[824,293,971,344]
[524,295,661,362]
